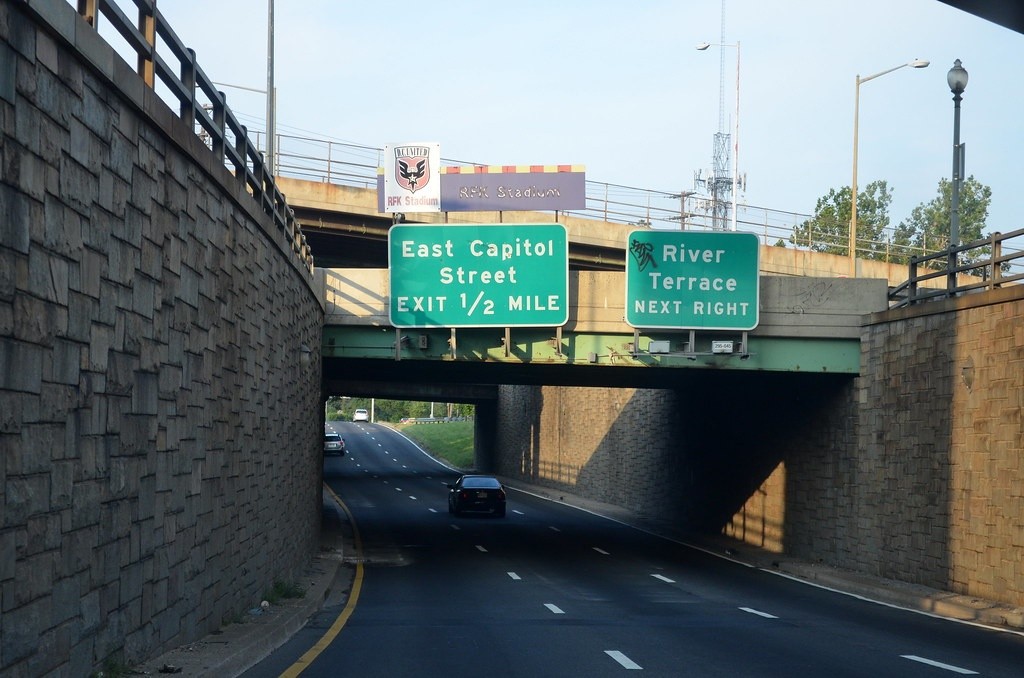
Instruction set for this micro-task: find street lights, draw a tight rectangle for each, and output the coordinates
[947,58,968,299]
[850,60,931,279]
[696,41,741,232]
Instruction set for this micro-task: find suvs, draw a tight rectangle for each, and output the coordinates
[325,433,346,454]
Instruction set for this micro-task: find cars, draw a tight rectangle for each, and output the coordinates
[447,475,507,520]
[354,409,369,422]
